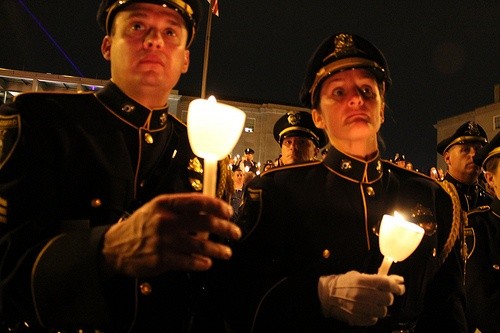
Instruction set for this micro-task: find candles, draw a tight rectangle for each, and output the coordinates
[191,94,218,257]
[377,210,405,275]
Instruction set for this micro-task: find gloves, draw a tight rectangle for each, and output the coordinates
[318,270,405,327]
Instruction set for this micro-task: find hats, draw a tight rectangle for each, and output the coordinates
[272,110,329,149]
[300,32,392,110]
[473,130,500,170]
[435,122,489,155]
[97,0,203,49]
[245,148,254,154]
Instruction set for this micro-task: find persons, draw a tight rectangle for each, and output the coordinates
[437,120,489,218]
[0,0,245,333]
[396,156,405,168]
[265,159,281,171]
[405,162,413,170]
[457,130,500,333]
[322,148,330,159]
[273,111,329,166]
[239,148,260,177]
[223,169,245,225]
[219,30,468,333]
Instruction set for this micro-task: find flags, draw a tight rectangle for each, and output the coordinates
[207,0,219,17]
